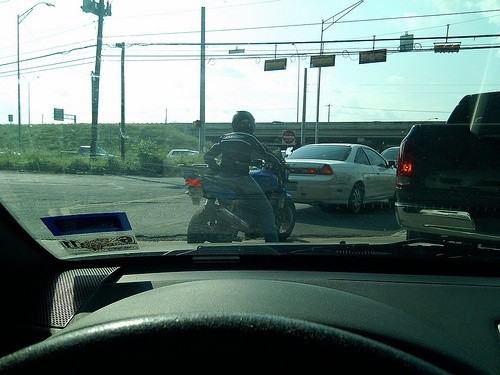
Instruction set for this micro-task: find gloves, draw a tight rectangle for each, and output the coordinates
[282,164,290,170]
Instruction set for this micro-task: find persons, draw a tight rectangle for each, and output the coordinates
[204,111,288,242]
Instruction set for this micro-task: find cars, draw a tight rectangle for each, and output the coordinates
[76,145,114,157]
[165,148,209,167]
[282,142,400,214]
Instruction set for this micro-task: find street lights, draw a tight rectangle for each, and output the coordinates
[17,2,55,145]
[291,42,300,122]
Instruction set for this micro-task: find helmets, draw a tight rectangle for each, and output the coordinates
[232,110,255,135]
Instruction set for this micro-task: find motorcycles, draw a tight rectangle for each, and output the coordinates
[180,147,296,243]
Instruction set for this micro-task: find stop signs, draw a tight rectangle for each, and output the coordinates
[282,130,295,144]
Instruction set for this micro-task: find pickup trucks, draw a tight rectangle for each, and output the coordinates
[393,90,500,245]
